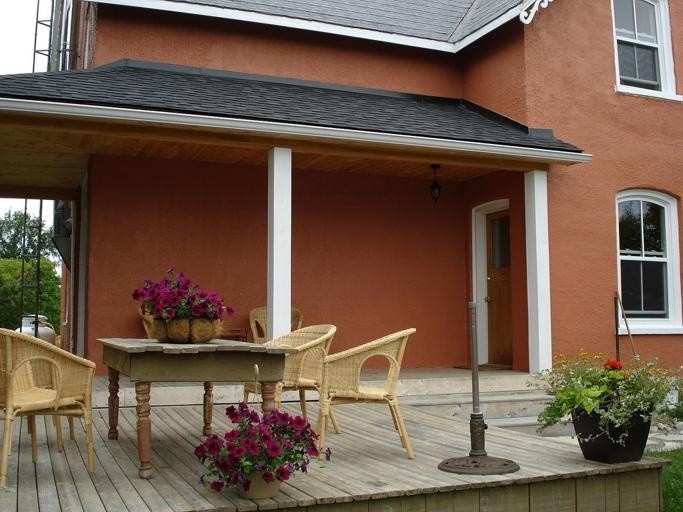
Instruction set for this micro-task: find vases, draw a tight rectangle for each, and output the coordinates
[138,308,222,343]
[236,470,281,499]
[570,404,653,463]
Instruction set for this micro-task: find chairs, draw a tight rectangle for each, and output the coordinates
[242,324,340,433]
[0,327,96,487]
[249,306,303,344]
[319,328,417,460]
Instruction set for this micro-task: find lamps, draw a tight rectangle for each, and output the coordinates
[429,163,442,203]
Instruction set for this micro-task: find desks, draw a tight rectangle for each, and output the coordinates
[95,337,298,479]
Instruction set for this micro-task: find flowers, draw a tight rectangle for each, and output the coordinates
[132,266,234,323]
[524,348,683,446]
[192,401,332,492]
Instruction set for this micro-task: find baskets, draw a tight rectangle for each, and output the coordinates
[152,314,221,342]
